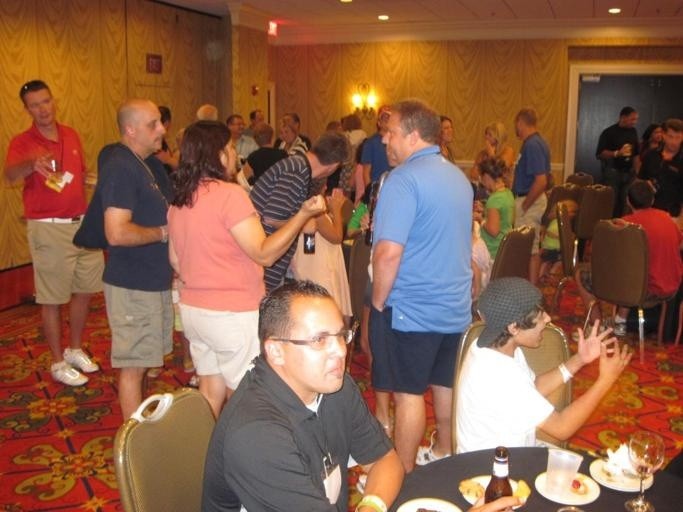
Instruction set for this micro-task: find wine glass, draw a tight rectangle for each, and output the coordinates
[623,429,666,510]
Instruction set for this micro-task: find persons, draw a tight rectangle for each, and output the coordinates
[101,99,174,423]
[152,100,392,421]
[4,81,99,386]
[455,277,634,455]
[198,279,404,512]
[438,105,683,343]
[367,99,475,472]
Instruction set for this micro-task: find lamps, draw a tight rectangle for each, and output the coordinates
[352,93,376,120]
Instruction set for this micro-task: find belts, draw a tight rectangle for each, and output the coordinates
[35,214,85,224]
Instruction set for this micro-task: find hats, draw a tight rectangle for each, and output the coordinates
[476,275,544,348]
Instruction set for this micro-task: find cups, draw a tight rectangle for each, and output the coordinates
[545,446,585,491]
[43,154,63,184]
[623,143,631,159]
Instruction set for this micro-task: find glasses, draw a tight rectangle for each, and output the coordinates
[271,326,356,354]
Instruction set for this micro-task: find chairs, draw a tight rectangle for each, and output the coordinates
[541,170,649,365]
[490,224,536,282]
[345,236,371,374]
[112,386,216,512]
[449,319,570,455]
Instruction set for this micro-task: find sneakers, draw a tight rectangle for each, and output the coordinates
[571,316,628,342]
[50,345,99,387]
[415,431,452,466]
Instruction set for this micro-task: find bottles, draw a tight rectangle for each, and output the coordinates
[365,196,374,243]
[302,213,317,253]
[484,446,513,510]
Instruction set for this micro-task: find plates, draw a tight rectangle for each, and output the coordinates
[534,472,603,506]
[589,454,655,494]
[457,475,529,509]
[394,496,462,511]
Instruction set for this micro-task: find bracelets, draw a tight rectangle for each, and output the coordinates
[355,495,388,512]
[559,362,574,383]
